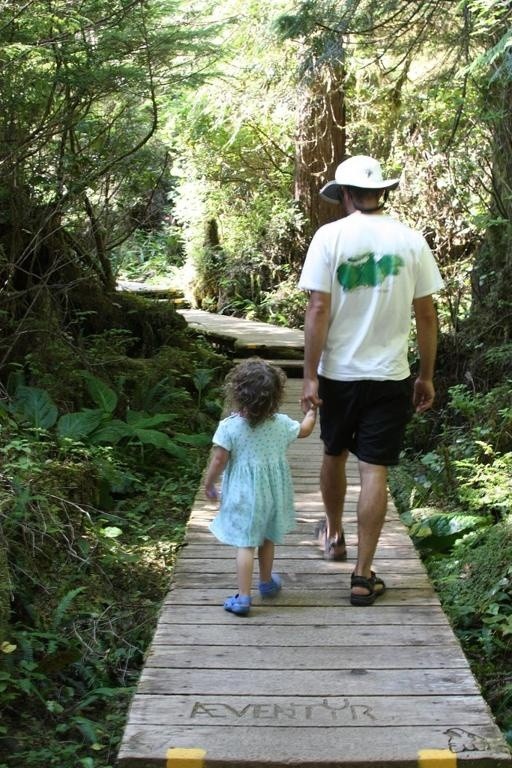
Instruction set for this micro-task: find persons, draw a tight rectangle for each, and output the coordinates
[299,154,447,606]
[202,355,318,615]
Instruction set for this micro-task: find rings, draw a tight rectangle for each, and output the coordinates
[309,403,314,408]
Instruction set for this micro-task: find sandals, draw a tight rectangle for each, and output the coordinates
[313,519,348,562]
[221,593,252,616]
[260,574,280,595]
[350,567,387,605]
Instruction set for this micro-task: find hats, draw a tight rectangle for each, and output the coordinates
[319,154,401,205]
